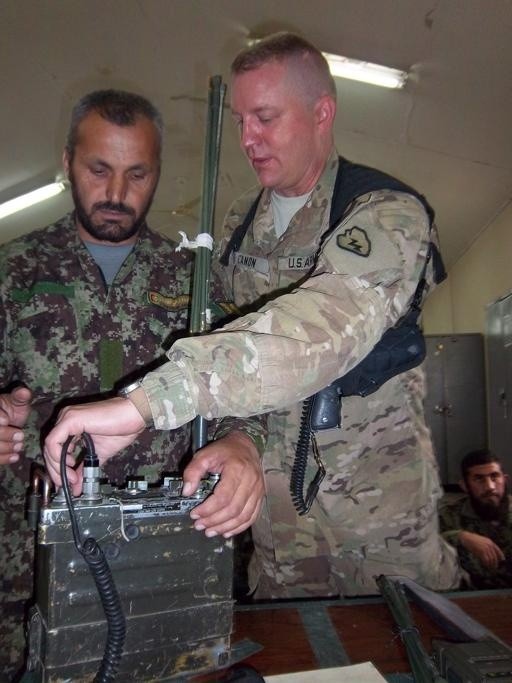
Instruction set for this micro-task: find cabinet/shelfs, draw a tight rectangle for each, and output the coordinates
[420,331,488,488]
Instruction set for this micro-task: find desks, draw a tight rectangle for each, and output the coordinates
[231,585,511,682]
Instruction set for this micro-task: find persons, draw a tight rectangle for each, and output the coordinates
[1,90,268,682]
[439,451,512,589]
[43,31,461,602]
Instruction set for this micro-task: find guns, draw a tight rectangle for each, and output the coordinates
[311,384,341,428]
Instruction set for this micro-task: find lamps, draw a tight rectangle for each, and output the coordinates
[321,50,408,91]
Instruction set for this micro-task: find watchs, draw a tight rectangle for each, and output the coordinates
[117,377,154,429]
